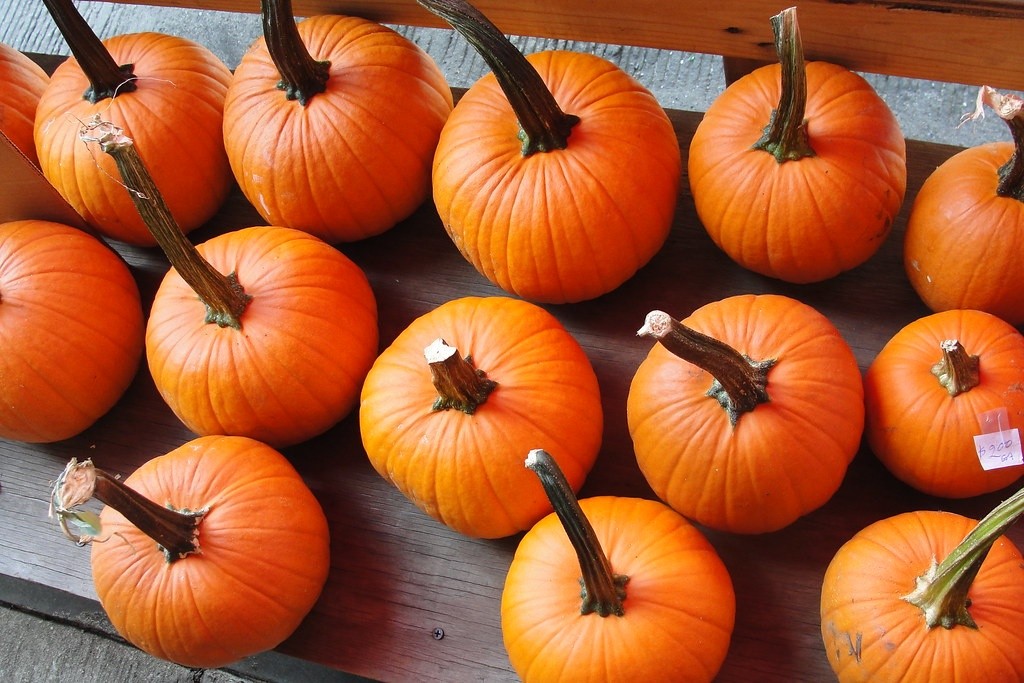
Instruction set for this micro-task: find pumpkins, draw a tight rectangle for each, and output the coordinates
[0,0,1024,683]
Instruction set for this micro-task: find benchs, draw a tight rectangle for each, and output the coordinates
[0,0,1024,683]
